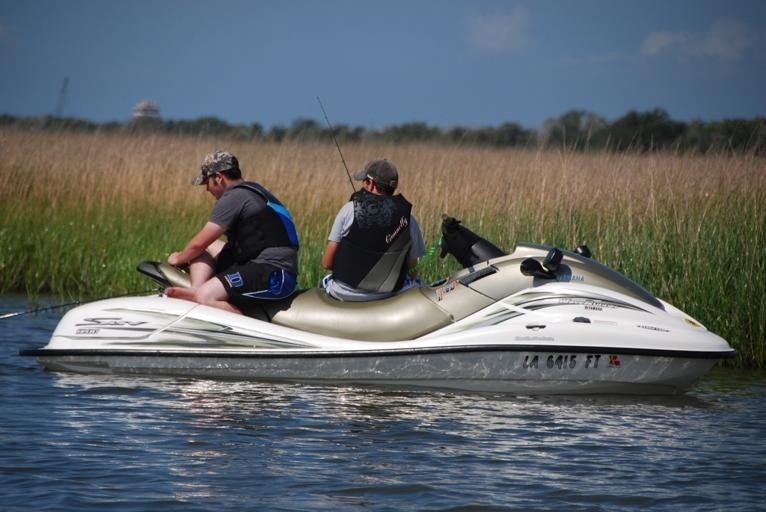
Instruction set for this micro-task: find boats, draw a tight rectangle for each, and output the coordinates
[17,211,741,397]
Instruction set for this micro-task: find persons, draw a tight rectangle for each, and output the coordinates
[162,150,299,315]
[319,158,427,302]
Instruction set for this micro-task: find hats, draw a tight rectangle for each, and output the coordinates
[194,149,239,186]
[352,158,399,190]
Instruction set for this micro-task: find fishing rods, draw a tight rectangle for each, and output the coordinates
[317,95,355,192]
[0,288,163,319]
